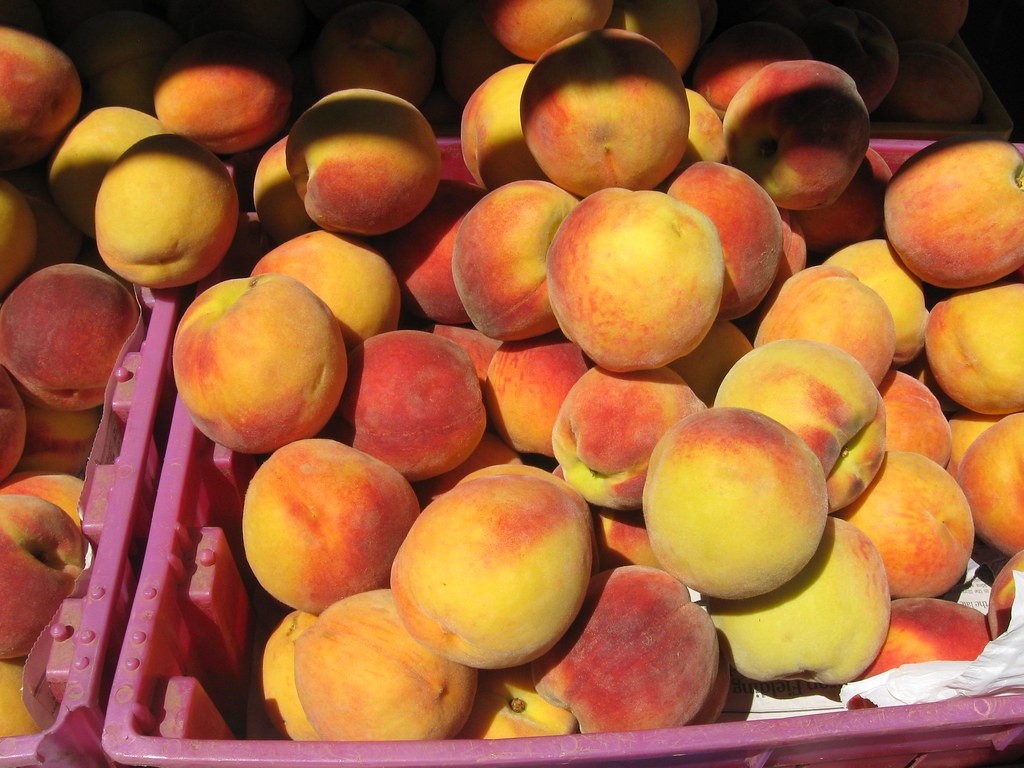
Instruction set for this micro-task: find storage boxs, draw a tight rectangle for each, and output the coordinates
[0,28,1023,768]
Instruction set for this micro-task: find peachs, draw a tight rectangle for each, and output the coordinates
[0,0,1024,741]
